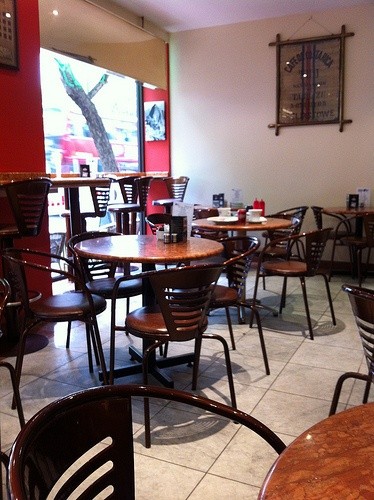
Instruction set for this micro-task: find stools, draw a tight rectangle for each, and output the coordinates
[60,180,113,293]
[152,176,190,213]
[0,177,54,357]
[107,175,153,273]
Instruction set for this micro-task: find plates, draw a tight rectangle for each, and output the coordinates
[246,217,267,224]
[207,217,238,223]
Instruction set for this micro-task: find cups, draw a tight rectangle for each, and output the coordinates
[238,209,246,225]
[218,208,231,217]
[156,230,168,240]
[247,209,262,220]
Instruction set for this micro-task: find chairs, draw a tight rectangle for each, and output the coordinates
[0,206,374,500]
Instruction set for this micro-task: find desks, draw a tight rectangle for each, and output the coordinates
[74,234,223,389]
[0,172,169,197]
[259,401,374,500]
[193,216,292,324]
[321,208,374,280]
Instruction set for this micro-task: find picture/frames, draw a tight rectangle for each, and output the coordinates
[0,0,19,71]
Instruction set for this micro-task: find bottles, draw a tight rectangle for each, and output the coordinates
[163,233,170,243]
[213,192,224,208]
[253,197,265,217]
[171,233,178,243]
